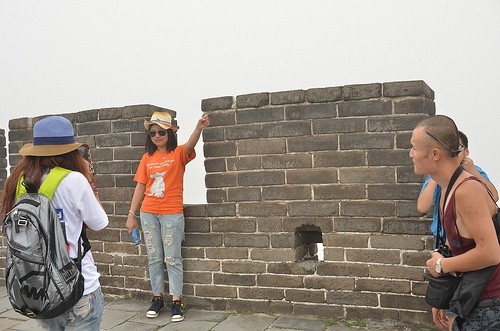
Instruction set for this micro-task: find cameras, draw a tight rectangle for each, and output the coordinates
[434,244,452,258]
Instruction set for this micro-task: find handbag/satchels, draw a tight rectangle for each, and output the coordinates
[426,274,461,310]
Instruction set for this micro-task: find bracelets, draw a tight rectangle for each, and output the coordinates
[128,210,137,218]
[88,178,99,196]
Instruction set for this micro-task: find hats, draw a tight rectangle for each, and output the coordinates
[144,112,180,132]
[19,116,89,156]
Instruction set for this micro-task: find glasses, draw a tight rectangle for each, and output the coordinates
[147,130,167,137]
[425,131,465,157]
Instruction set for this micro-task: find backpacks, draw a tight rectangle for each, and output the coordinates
[2,166,91,320]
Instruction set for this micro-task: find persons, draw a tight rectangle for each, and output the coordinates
[0,116,109,331]
[124,110,209,322]
[407,114,500,331]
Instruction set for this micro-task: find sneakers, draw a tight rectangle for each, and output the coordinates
[172,304,185,322]
[146,293,164,319]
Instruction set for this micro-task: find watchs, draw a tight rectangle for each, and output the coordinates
[435,258,444,275]
